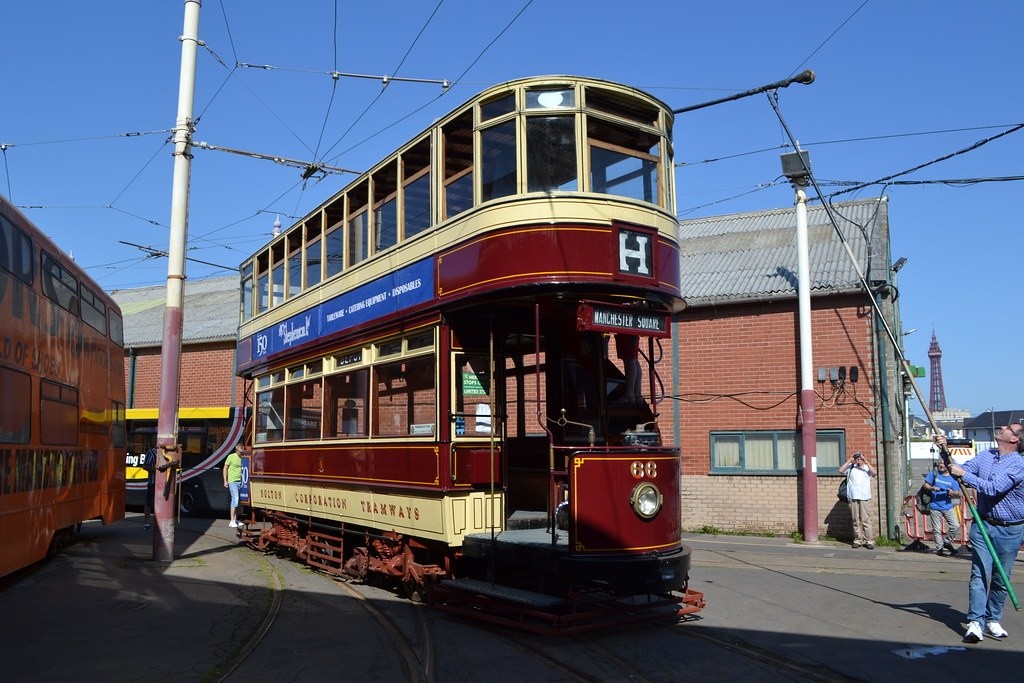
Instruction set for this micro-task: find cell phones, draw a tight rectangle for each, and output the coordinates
[854,454,860,458]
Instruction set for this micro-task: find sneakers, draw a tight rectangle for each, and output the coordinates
[964,620,984,641]
[985,621,1008,637]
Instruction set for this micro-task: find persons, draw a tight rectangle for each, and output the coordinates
[839,453,876,549]
[933,422,1024,641]
[223,442,245,528]
[924,459,962,555]
[143,448,157,532]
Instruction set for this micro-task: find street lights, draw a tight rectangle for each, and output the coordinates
[781,148,820,542]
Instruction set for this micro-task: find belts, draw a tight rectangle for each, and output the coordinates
[983,515,1024,528]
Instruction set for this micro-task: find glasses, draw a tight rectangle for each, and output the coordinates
[1006,424,1018,437]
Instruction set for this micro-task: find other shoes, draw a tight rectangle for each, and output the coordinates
[144,524,151,532]
[943,542,957,553]
[937,548,943,556]
[229,521,238,527]
[235,519,244,526]
[867,544,874,549]
[852,544,861,548]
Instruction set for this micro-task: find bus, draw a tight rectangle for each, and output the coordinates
[0,197,129,589]
[123,408,240,517]
[233,74,704,637]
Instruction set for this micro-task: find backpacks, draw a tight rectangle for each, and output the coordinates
[837,477,848,502]
[915,469,937,515]
